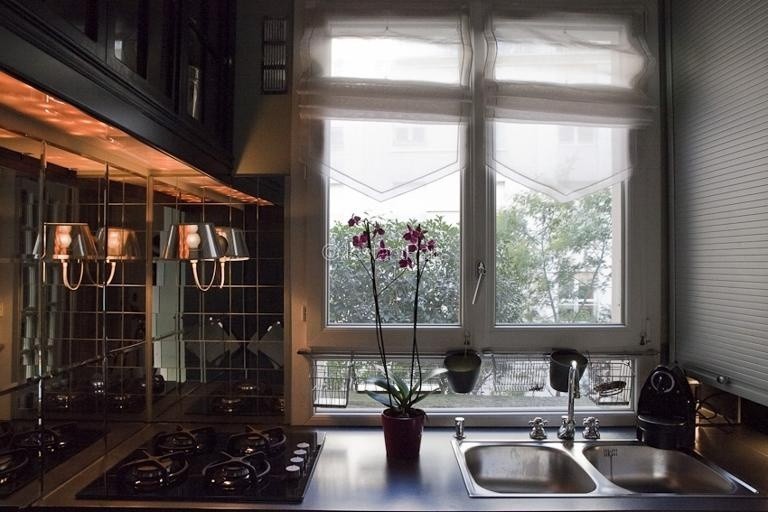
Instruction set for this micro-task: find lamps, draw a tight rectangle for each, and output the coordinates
[33,222,99,291]
[215,226,250,288]
[161,220,222,293]
[85,228,144,288]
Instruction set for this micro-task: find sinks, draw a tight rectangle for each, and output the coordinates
[579,436,760,497]
[449,437,603,495]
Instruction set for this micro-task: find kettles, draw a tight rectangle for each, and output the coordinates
[635,359,698,453]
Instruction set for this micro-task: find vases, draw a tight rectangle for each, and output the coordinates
[444,351,481,393]
[549,351,588,393]
[381,408,425,459]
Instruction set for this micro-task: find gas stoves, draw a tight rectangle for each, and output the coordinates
[0,421,103,497]
[79,424,324,503]
[186,379,285,416]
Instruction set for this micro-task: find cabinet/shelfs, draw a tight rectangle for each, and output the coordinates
[0,1,238,185]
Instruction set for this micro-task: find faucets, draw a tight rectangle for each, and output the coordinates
[557,359,578,438]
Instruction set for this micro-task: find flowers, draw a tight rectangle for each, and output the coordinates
[346,213,448,414]
